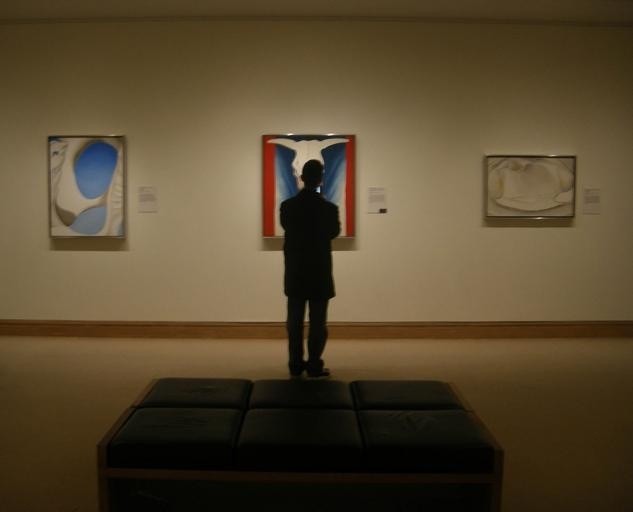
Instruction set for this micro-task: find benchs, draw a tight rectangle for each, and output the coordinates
[97,377,504,512]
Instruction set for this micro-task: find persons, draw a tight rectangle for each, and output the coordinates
[279,158,341,376]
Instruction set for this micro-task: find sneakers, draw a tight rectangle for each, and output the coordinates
[307,368,330,377]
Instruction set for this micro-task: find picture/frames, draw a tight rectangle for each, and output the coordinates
[484,155,576,219]
[46,135,127,240]
